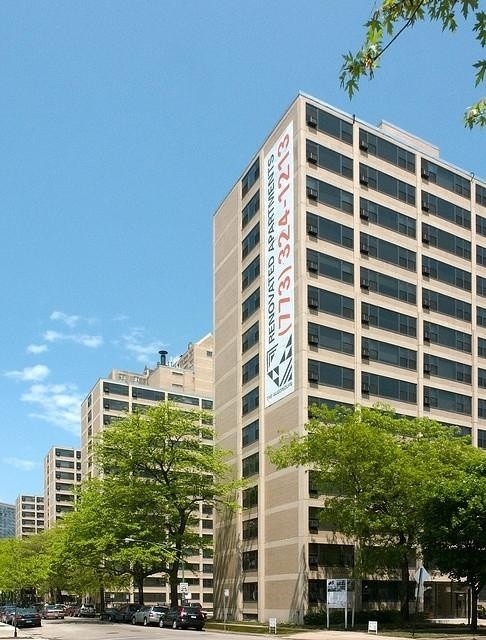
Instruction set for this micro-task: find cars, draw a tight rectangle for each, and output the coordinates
[0,601,208,632]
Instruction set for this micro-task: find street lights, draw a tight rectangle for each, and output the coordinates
[121,537,188,608]
